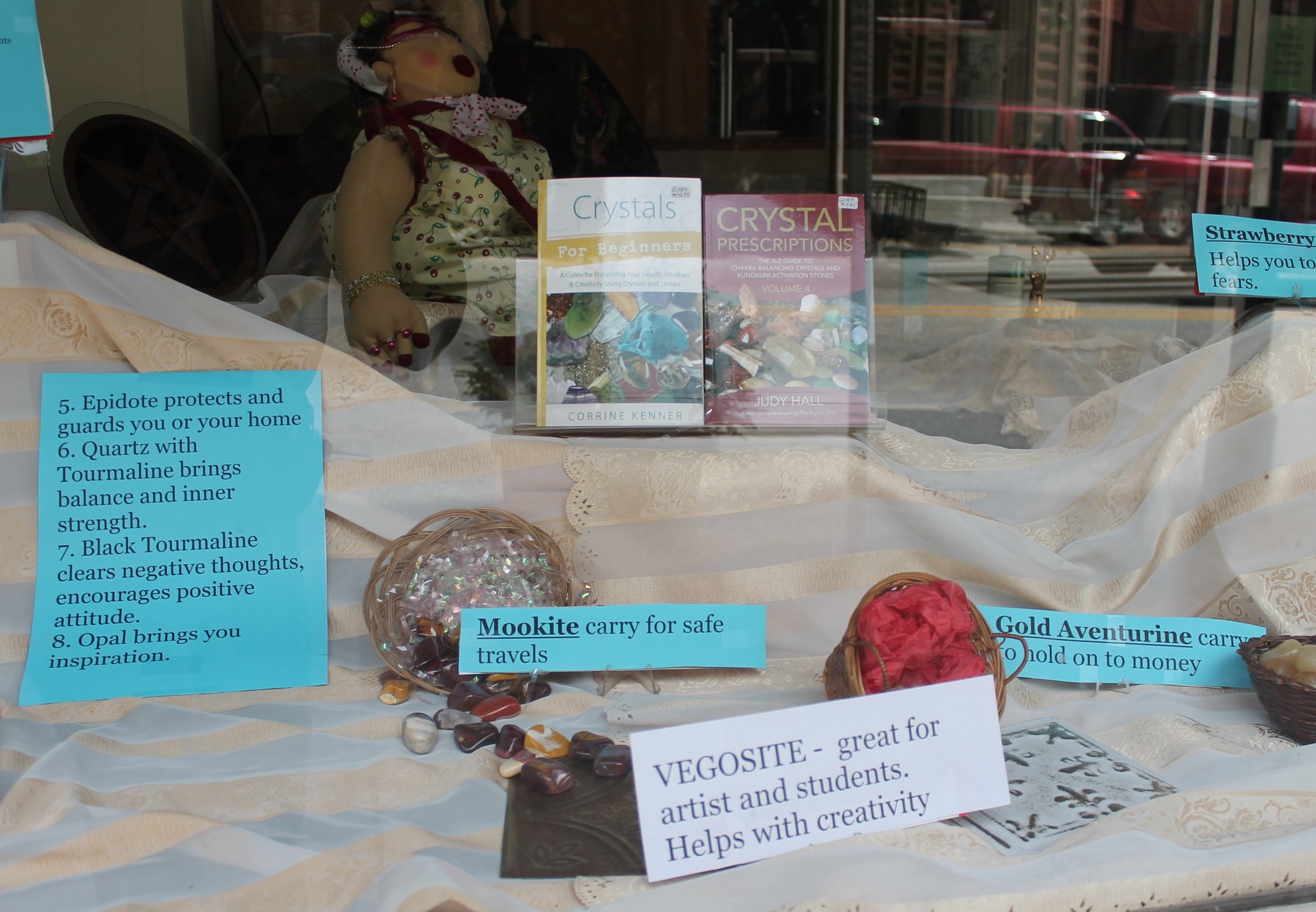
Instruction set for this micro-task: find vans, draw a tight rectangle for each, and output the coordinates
[1124,88,1316,224]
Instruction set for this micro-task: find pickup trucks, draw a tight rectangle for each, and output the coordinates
[867,107,1193,244]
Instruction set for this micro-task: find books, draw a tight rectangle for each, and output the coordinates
[537,177,705,429]
[704,193,871,428]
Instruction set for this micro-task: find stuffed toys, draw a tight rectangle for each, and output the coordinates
[316,6,553,381]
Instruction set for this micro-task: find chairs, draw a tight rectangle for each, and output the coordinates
[49,103,272,305]
